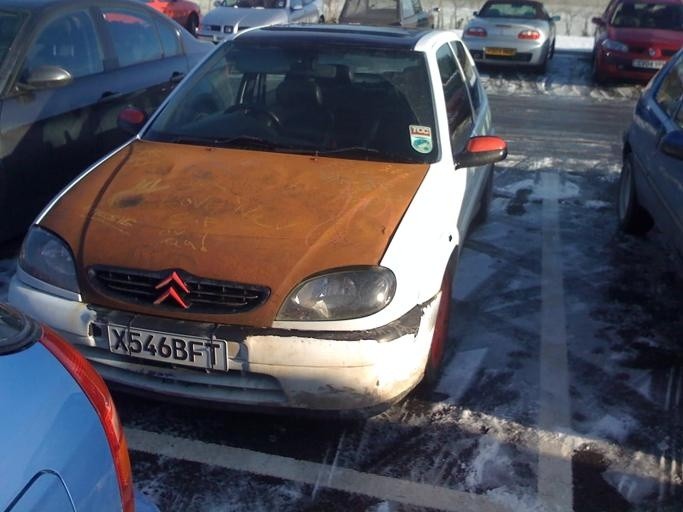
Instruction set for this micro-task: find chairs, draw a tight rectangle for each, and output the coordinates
[49,17,82,70]
[268,61,420,149]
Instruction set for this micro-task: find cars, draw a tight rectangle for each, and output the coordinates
[615,47,682,254]
[590,0,683,86]
